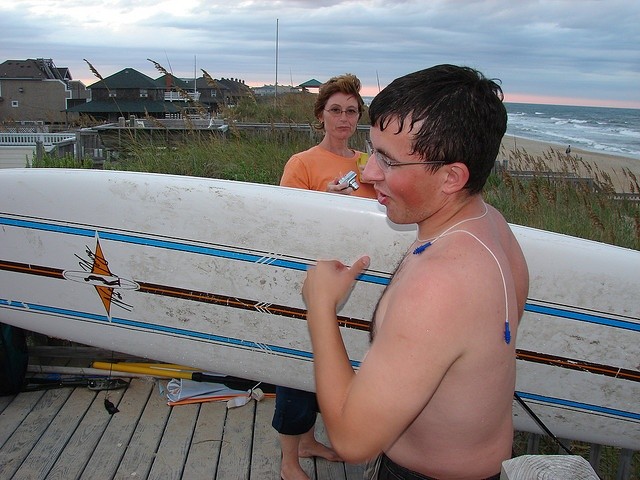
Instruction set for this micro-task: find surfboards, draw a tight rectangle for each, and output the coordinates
[0,166,639,454]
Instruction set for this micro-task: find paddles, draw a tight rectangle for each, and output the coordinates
[93,361,277,395]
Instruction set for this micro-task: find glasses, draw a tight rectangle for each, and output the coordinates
[323,105,365,119]
[365,138,454,173]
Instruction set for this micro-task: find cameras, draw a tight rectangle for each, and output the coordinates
[338,171,360,190]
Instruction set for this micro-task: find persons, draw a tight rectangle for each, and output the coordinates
[272,73,380,480]
[300,65,530,479]
